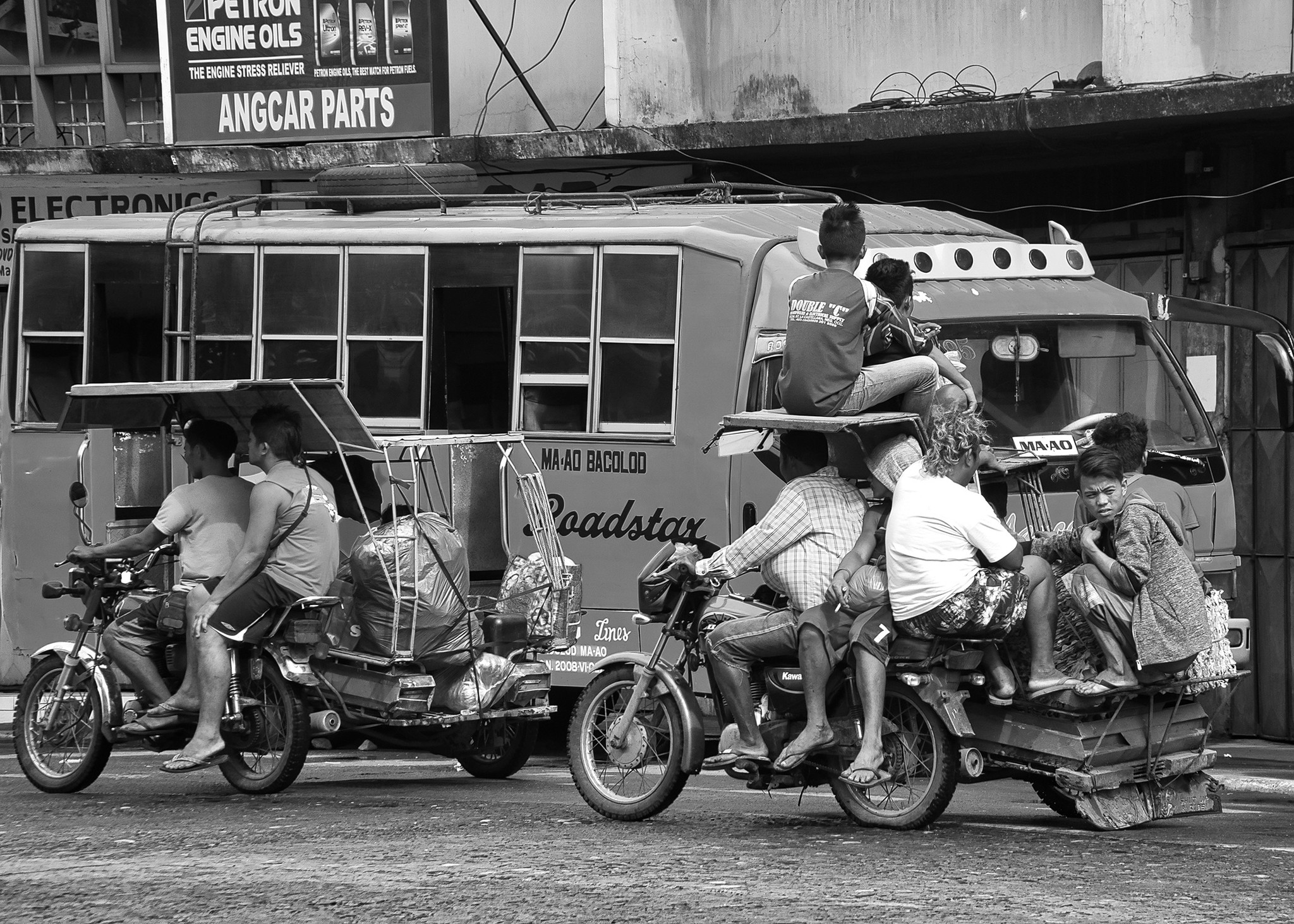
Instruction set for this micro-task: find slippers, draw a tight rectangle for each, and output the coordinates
[1027,676,1077,701]
[773,737,840,773]
[159,753,230,773]
[838,762,893,789]
[147,703,199,717]
[115,718,187,735]
[701,748,772,771]
[984,681,1018,706]
[1138,678,1172,685]
[1072,676,1143,697]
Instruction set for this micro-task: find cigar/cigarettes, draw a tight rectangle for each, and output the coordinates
[934,327,939,331]
[834,601,842,615]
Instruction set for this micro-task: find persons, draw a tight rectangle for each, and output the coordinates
[146,405,339,773]
[675,430,870,769]
[772,434,924,789]
[339,503,426,585]
[774,200,939,432]
[66,419,256,734]
[1020,444,1214,698]
[978,480,1027,542]
[884,400,1085,706]
[863,259,978,415]
[1034,412,1199,564]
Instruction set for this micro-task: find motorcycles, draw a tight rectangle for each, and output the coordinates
[567,542,985,828]
[10,479,338,794]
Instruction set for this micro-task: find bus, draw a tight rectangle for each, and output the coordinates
[0,190,1293,758]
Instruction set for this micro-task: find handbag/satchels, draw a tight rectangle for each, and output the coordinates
[494,552,583,652]
[318,577,363,653]
